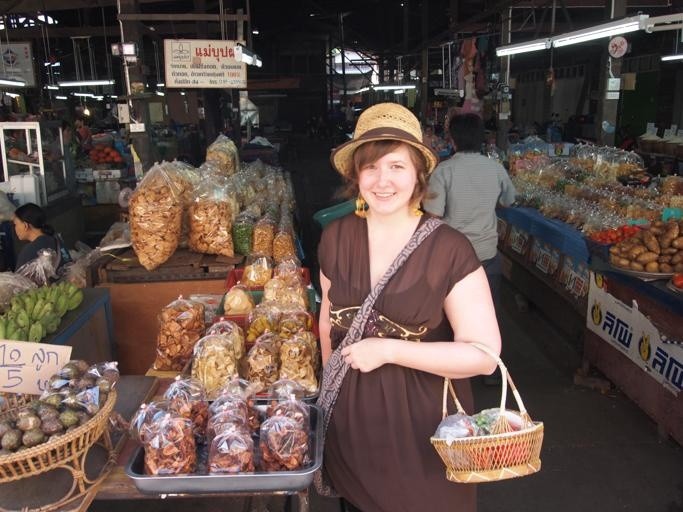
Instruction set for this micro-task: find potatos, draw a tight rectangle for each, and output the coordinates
[609,216,683,274]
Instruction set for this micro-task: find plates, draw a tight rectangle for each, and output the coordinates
[607,263,677,279]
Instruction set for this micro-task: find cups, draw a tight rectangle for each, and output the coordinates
[9,174,40,209]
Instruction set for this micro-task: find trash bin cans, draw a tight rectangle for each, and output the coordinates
[92,169,136,205]
[313,198,366,229]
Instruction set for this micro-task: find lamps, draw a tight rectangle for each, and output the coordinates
[0,0,264,117]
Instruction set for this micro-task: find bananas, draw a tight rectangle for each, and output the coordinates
[0,281,83,342]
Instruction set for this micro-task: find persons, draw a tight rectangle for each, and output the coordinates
[424,125,438,146]
[54,119,80,168]
[11,201,64,271]
[313,101,508,511]
[422,110,518,301]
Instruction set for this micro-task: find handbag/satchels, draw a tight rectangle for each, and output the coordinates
[315,347,347,498]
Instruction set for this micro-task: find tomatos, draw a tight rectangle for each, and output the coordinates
[672,273,683,288]
[590,225,641,244]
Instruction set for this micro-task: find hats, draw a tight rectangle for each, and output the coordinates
[330,102,439,182]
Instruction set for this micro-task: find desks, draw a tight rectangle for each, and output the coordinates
[0,375,159,512]
[91,374,313,512]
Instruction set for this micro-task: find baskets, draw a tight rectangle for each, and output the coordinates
[430,343,545,484]
